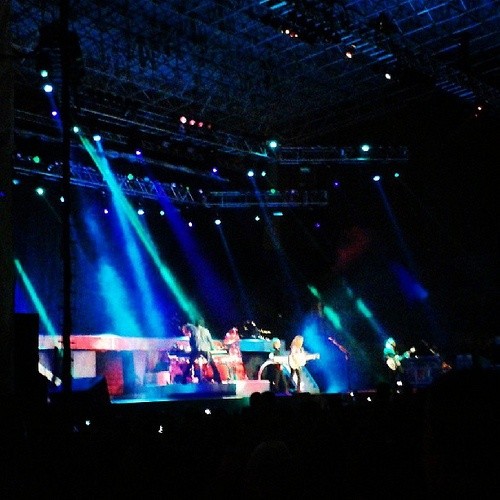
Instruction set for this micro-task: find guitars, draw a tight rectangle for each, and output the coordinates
[288,352,320,370]
[386,346,416,371]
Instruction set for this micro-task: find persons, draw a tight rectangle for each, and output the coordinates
[223,328,239,353]
[181,319,222,384]
[270,337,291,395]
[288,335,316,393]
[0,374,500,500]
[383,338,405,383]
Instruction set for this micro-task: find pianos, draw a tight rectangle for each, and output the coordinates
[168,348,229,355]
